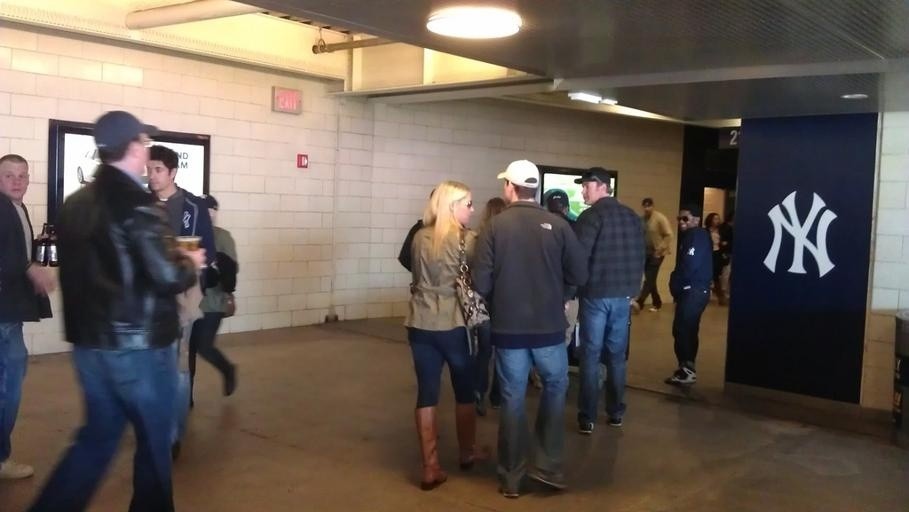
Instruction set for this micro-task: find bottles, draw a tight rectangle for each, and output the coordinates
[34,223,60,267]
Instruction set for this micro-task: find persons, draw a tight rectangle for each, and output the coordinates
[0,154,60,481]
[631,197,674,313]
[24,111,238,512]
[663,203,732,385]
[399,158,647,500]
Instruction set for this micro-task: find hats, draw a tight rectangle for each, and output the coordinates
[201,194,216,205]
[94,110,160,150]
[548,190,569,208]
[574,168,610,186]
[496,160,541,190]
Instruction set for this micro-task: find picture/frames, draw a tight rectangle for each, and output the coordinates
[47,119,211,225]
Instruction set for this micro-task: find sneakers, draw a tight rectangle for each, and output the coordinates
[631,300,660,312]
[525,470,568,490]
[580,421,593,434]
[607,416,623,427]
[501,490,520,498]
[665,367,697,385]
[0,457,33,480]
[473,387,501,416]
[225,364,235,395]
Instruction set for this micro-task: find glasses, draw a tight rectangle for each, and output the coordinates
[462,197,474,210]
[677,215,690,222]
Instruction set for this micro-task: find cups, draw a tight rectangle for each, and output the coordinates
[176,235,202,251]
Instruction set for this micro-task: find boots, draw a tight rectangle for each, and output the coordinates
[456,400,492,468]
[415,403,448,490]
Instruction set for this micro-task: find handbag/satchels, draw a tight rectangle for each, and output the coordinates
[454,271,492,329]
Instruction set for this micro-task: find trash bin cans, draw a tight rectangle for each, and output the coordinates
[893,311,908,446]
